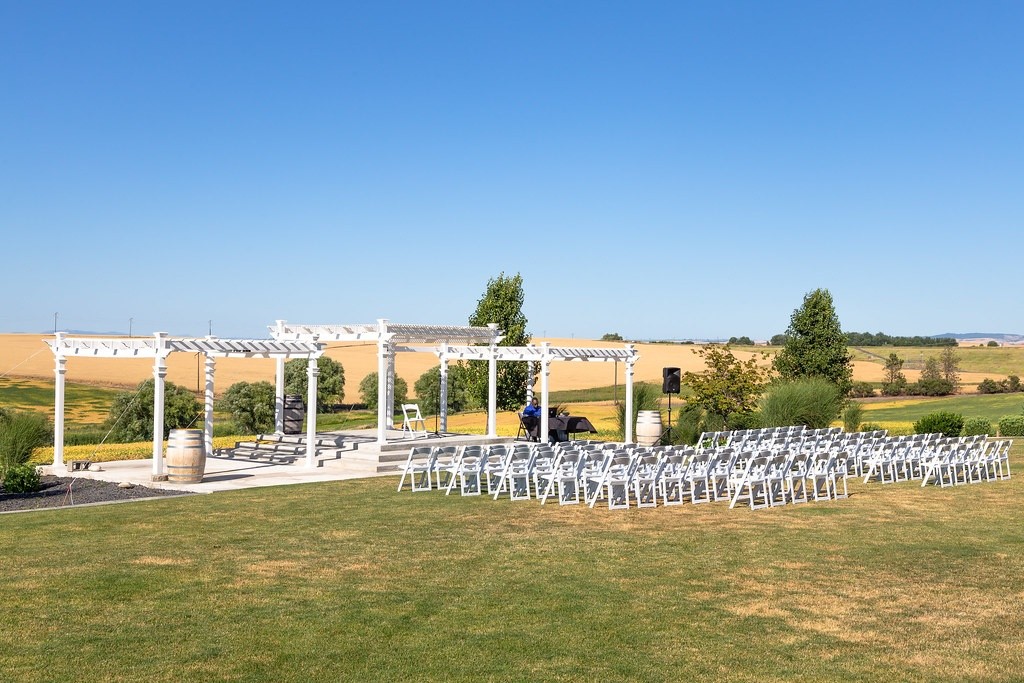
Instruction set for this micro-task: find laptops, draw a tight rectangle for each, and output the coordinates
[548,407,557,418]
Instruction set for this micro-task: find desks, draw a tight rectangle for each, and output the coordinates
[523,416,597,443]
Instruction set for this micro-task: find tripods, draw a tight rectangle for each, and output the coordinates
[651,393,684,447]
[429,389,446,439]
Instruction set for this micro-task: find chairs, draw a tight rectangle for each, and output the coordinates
[399,425,1015,511]
[401,403,429,439]
[518,412,541,442]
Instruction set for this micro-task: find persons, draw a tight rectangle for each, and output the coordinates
[522,397,541,441]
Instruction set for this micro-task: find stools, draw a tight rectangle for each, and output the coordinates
[67,460,92,472]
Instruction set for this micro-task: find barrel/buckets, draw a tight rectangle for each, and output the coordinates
[637,411,662,447]
[167,428,207,485]
[282,394,304,433]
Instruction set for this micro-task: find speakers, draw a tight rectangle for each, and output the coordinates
[663,368,680,394]
